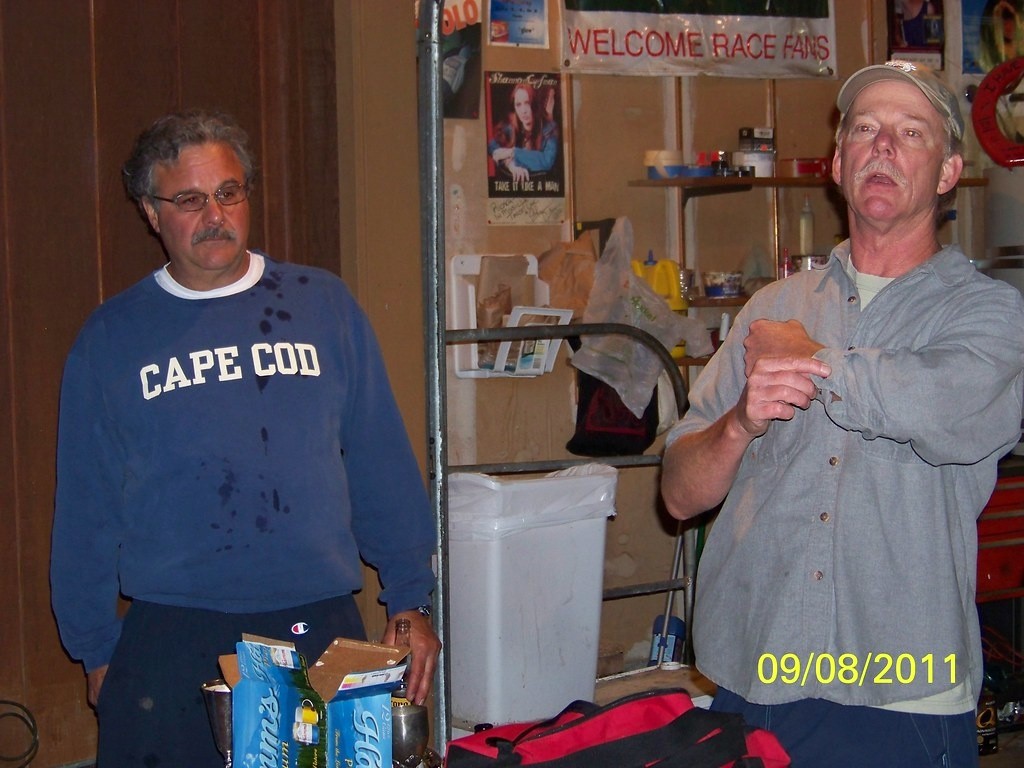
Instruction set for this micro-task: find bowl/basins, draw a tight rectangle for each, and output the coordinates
[700,271,743,299]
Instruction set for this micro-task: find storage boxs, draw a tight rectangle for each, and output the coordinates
[737,127,775,153]
[216,632,413,768]
[780,157,832,178]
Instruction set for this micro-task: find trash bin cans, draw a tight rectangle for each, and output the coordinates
[430,460,619,726]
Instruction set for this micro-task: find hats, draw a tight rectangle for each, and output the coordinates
[836,60,964,142]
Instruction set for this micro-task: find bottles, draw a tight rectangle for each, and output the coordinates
[799,194,814,256]
[633,249,689,358]
[779,248,793,280]
[391,618,415,709]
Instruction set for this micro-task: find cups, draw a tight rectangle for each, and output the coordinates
[392,706,429,768]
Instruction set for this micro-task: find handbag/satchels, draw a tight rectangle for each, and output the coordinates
[566,215,715,458]
[445,687,791,768]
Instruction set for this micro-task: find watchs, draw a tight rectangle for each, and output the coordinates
[419,607,431,616]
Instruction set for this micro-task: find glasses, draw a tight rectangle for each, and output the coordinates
[154,177,250,213]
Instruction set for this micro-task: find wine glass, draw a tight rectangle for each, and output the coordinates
[203,680,233,768]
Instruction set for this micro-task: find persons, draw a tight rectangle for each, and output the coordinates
[662,60,1024,768]
[490,82,558,180]
[50,110,442,768]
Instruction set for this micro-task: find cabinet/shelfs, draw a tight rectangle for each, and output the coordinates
[627,176,992,369]
